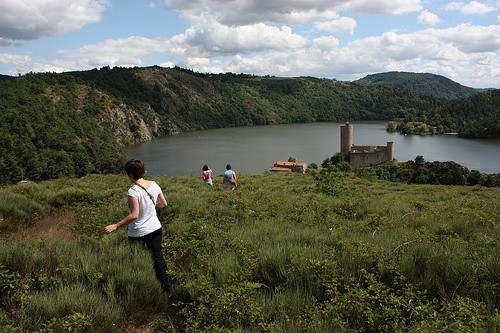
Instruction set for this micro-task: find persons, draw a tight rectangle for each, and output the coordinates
[202,165,214,186]
[102,159,173,298]
[223,164,237,190]
